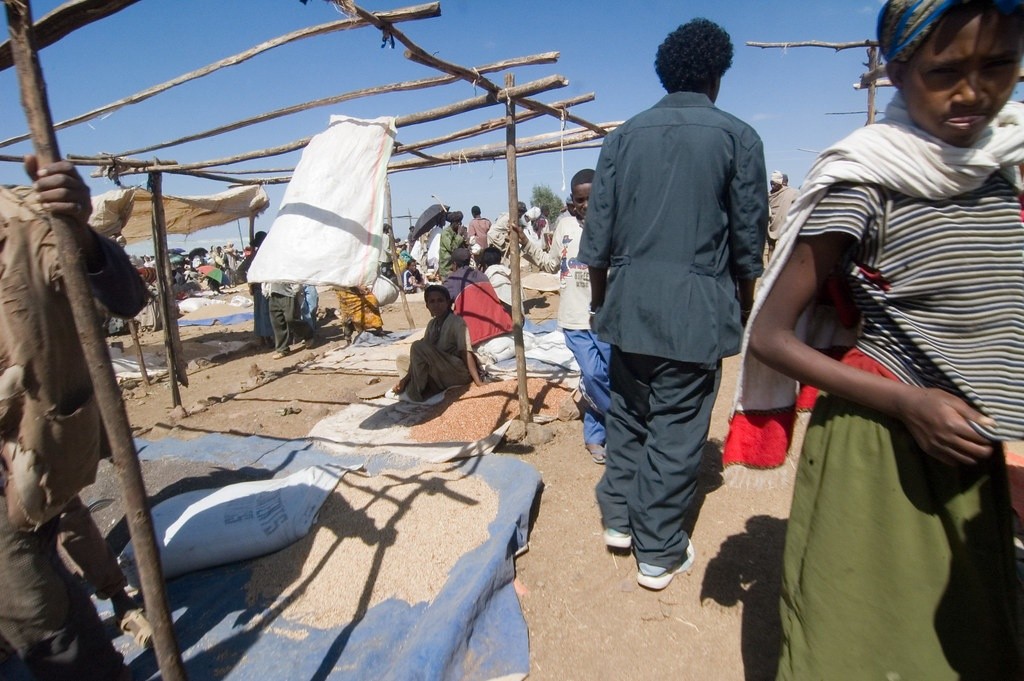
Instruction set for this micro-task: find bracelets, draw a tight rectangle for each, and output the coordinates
[86,268,104,277]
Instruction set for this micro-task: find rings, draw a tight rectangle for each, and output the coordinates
[77,203,81,213]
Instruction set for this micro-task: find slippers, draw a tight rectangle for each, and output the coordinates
[589,445,607,464]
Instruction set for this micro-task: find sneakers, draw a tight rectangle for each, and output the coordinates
[605,527,632,548]
[635,538,695,590]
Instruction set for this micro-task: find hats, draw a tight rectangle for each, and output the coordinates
[782,174,789,180]
[771,170,783,184]
[452,248,473,266]
[445,212,463,222]
[566,195,574,205]
[876,0,954,73]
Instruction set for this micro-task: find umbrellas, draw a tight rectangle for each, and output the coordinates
[197,265,231,289]
[412,203,450,241]
[167,247,186,254]
[188,247,207,260]
[168,254,184,267]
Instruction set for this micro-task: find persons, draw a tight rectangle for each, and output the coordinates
[442,245,525,347]
[379,203,553,291]
[238,231,319,359]
[395,290,489,404]
[506,168,614,464]
[577,16,769,594]
[335,283,388,344]
[129,241,251,332]
[766,169,799,266]
[0,153,149,681]
[720,0,1024,681]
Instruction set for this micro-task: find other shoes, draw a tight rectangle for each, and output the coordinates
[305,337,314,348]
[273,350,290,359]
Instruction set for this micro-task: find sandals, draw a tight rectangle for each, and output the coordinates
[117,608,153,649]
